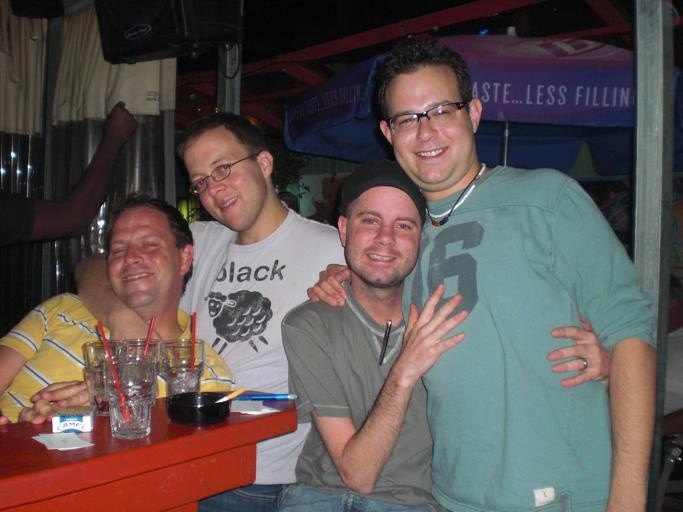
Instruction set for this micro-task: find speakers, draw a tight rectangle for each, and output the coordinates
[93,0,244,65]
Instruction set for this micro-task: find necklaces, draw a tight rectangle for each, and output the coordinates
[426,164,481,227]
[425,162,485,217]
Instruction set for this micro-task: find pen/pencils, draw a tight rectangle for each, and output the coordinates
[378,320,393,366]
[235,394,298,401]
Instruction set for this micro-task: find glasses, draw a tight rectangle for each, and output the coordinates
[387,102,467,131]
[188,153,257,196]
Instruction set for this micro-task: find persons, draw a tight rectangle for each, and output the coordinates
[1,101,137,249]
[307,42,656,511]
[0,193,234,424]
[76,114,349,512]
[275,159,467,512]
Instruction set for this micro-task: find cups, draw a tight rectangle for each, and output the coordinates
[102,354,156,440]
[80,338,204,418]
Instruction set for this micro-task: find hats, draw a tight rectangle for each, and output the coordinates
[338,159,426,226]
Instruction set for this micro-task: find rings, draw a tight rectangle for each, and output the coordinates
[576,357,587,371]
